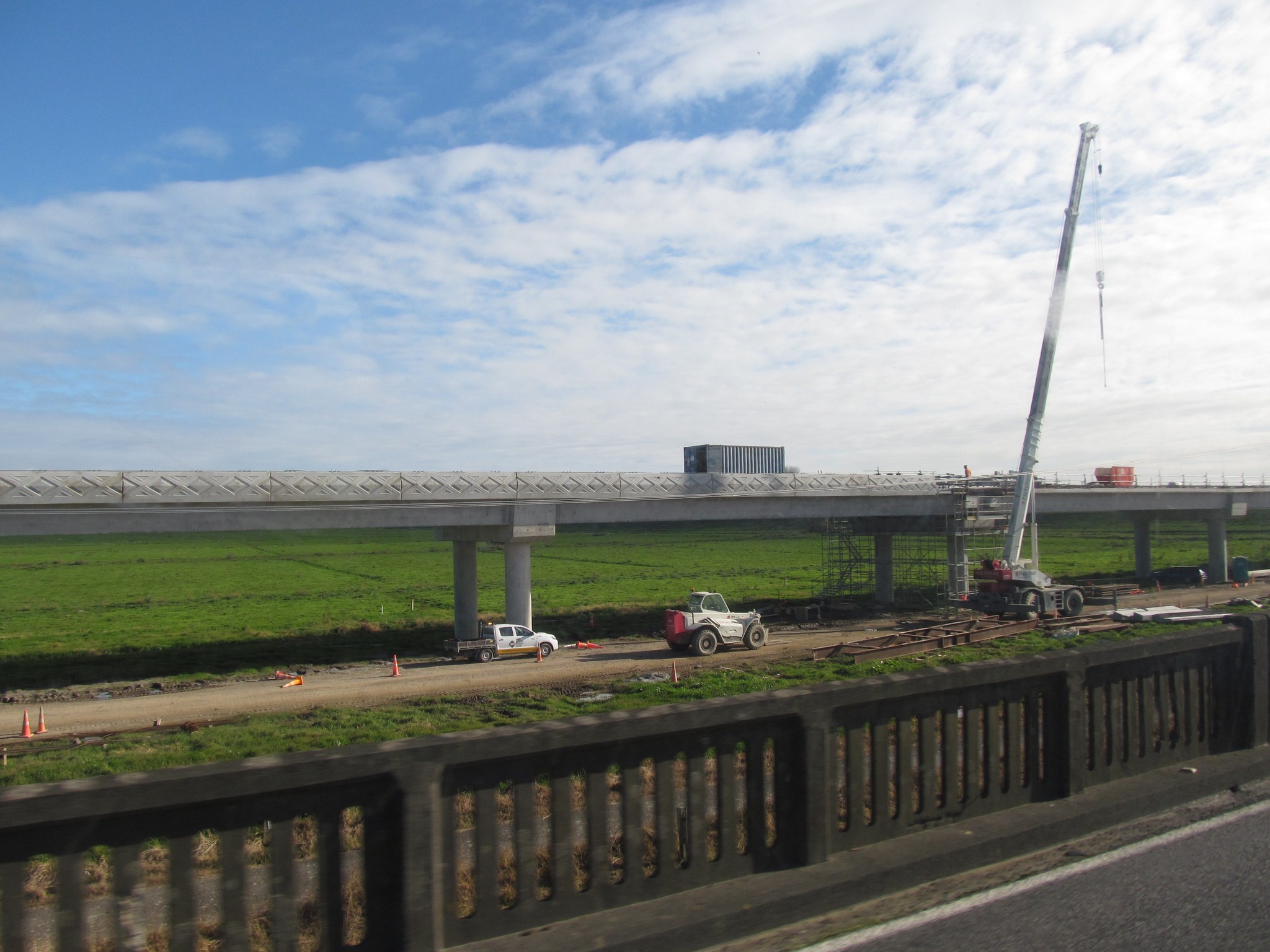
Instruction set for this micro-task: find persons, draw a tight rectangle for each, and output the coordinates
[963,465,972,487]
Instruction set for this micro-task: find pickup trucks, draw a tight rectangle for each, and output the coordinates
[443,621,559,663]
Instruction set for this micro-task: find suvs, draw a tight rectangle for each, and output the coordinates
[1151,565,1207,586]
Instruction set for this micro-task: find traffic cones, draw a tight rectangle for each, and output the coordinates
[1155,580,1162,591]
[34,705,48,734]
[670,661,678,683]
[275,671,296,680]
[390,655,401,676]
[576,641,587,650]
[586,641,605,649]
[534,642,545,663]
[282,675,303,688]
[18,709,34,738]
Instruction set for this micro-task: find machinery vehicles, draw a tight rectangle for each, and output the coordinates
[949,111,1110,623]
[665,588,770,657]
